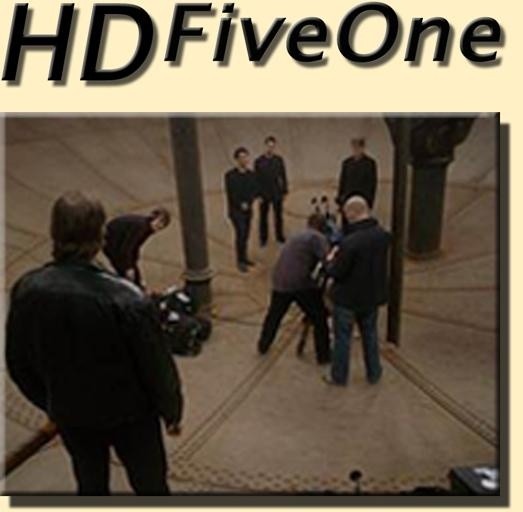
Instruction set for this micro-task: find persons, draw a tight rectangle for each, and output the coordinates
[334,138,377,229]
[321,194,394,386]
[255,137,288,245]
[102,206,170,290]
[224,148,264,273]
[256,213,333,364]
[4,190,183,496]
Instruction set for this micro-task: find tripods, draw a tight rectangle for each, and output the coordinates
[296,264,330,357]
